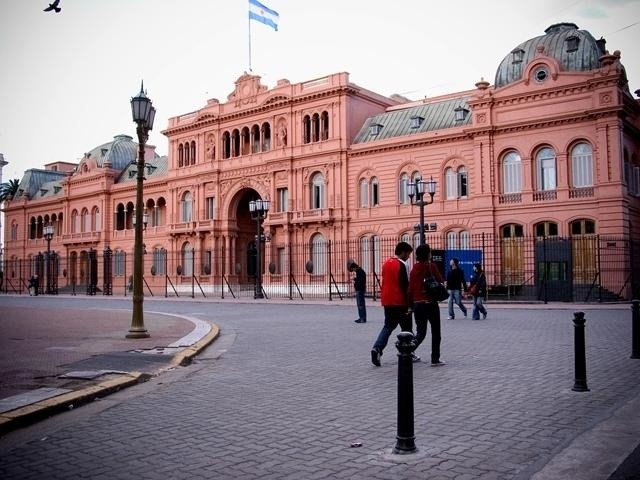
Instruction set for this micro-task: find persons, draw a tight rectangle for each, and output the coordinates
[468,264,488,319]
[409,245,446,368]
[446,258,468,320]
[370,242,420,368]
[347,263,366,324]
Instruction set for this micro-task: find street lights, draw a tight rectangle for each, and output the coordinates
[407,174,436,245]
[126,79,157,338]
[129,210,150,232]
[248,199,271,299]
[42,224,55,294]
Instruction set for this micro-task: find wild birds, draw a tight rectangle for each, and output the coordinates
[42,0,61,12]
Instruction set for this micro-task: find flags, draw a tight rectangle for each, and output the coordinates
[248,0,281,31]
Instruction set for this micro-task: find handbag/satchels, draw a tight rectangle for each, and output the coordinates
[469,285,476,294]
[426,281,450,302]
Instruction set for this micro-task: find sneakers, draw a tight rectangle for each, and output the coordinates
[371,348,382,366]
[412,350,421,362]
[431,359,444,365]
